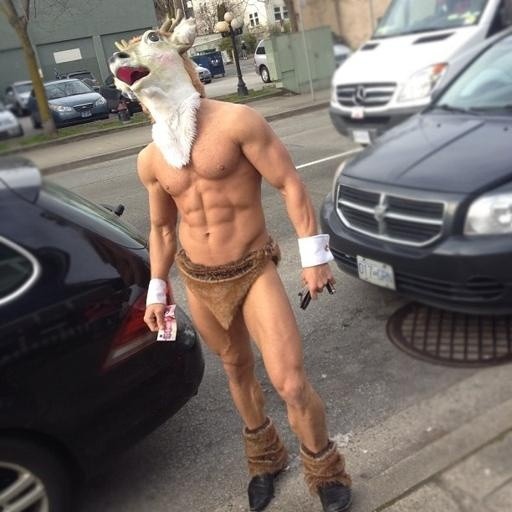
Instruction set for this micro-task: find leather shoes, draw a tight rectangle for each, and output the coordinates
[315,483,352,512]
[247,469,277,511]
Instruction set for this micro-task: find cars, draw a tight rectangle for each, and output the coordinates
[1,153,204,512]
[0,59,141,136]
[318,28,511,317]
[192,60,212,82]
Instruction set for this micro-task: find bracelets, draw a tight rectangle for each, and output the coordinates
[145,278,169,306]
[296,233,335,268]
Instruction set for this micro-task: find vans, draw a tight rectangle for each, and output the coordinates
[252,35,353,83]
[328,1,511,152]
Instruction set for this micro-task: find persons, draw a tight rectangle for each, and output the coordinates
[448,1,474,26]
[106,9,353,512]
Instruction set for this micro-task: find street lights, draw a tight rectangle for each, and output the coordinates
[216,10,252,97]
